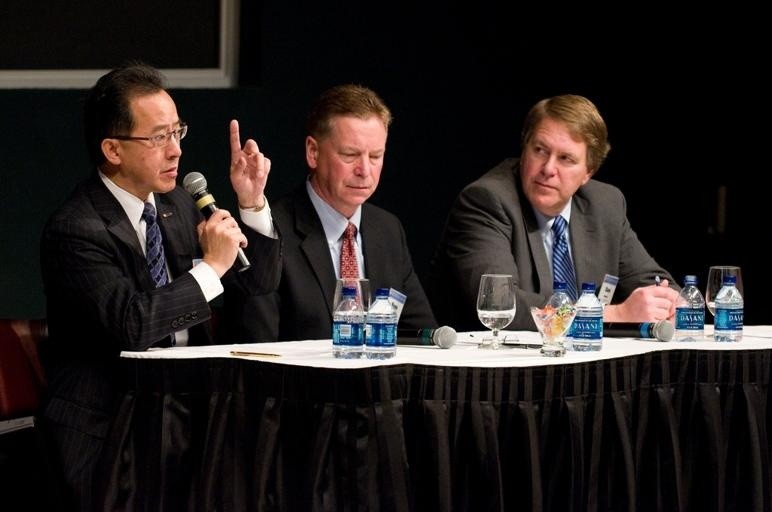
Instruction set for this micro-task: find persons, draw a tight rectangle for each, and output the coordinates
[270,81,439,342]
[426,93,690,331]
[40,57,283,511]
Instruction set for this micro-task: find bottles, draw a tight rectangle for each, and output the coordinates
[545,280,576,348]
[572,283,604,351]
[333,288,399,360]
[673,272,706,341]
[712,276,746,342]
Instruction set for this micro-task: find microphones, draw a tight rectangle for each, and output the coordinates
[182,172,251,273]
[363,325,457,349]
[603,319,675,342]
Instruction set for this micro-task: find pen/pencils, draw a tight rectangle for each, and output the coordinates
[230,350,281,356]
[655,275,661,286]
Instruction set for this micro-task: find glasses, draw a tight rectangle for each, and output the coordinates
[109,122,188,147]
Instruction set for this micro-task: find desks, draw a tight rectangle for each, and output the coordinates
[104,323,772,512]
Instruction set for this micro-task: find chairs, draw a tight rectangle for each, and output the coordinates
[0,318,41,418]
[13,318,48,387]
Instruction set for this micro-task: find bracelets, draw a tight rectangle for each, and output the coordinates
[238,200,266,212]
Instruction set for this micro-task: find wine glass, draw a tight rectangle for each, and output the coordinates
[705,263,744,317]
[475,272,518,350]
[527,306,576,356]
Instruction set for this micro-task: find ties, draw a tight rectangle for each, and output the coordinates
[142,202,175,288]
[340,222,361,304]
[552,215,577,303]
